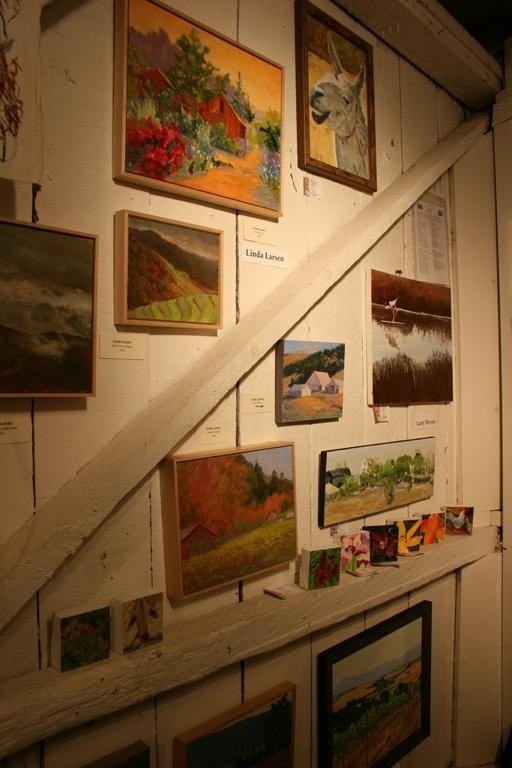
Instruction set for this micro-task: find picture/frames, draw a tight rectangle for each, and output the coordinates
[318,600,431,768]
[172,682,296,768]
[82,741,151,766]
[113,0,285,218]
[294,0,377,193]
[113,210,224,330]
[0,217,99,398]
[160,440,298,601]
